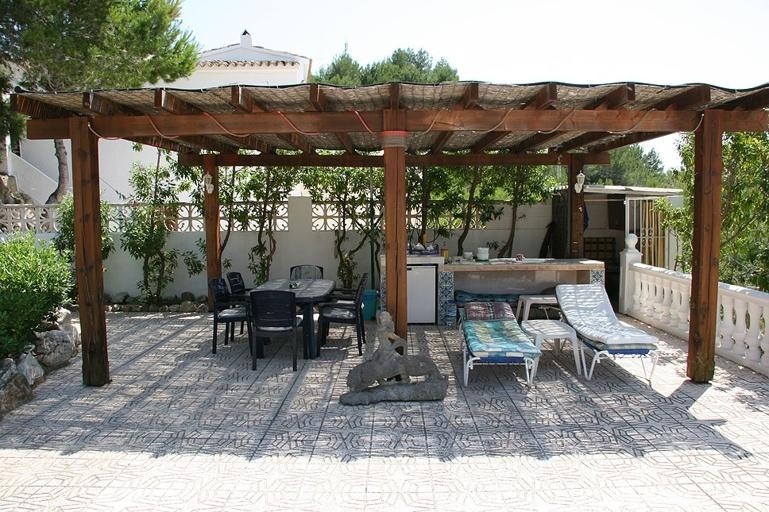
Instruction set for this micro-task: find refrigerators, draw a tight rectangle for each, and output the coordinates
[407,266,437,325]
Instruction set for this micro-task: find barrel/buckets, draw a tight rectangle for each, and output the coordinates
[361,290,377,321]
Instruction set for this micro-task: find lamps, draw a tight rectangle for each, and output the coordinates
[574,170,585,194]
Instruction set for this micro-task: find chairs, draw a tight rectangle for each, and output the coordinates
[556,280,660,382]
[457,301,540,384]
[207,264,370,371]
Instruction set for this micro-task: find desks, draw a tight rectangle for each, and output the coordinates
[437,257,606,326]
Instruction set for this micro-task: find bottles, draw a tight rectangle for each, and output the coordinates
[442,238,449,264]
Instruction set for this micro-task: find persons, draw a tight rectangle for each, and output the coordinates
[374,309,411,384]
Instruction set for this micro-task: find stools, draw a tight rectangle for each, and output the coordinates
[523,318,582,381]
[516,295,557,324]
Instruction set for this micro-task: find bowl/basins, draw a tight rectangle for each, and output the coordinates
[477,248,490,260]
[463,251,472,259]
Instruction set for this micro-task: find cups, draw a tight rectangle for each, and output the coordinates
[517,253,525,263]
